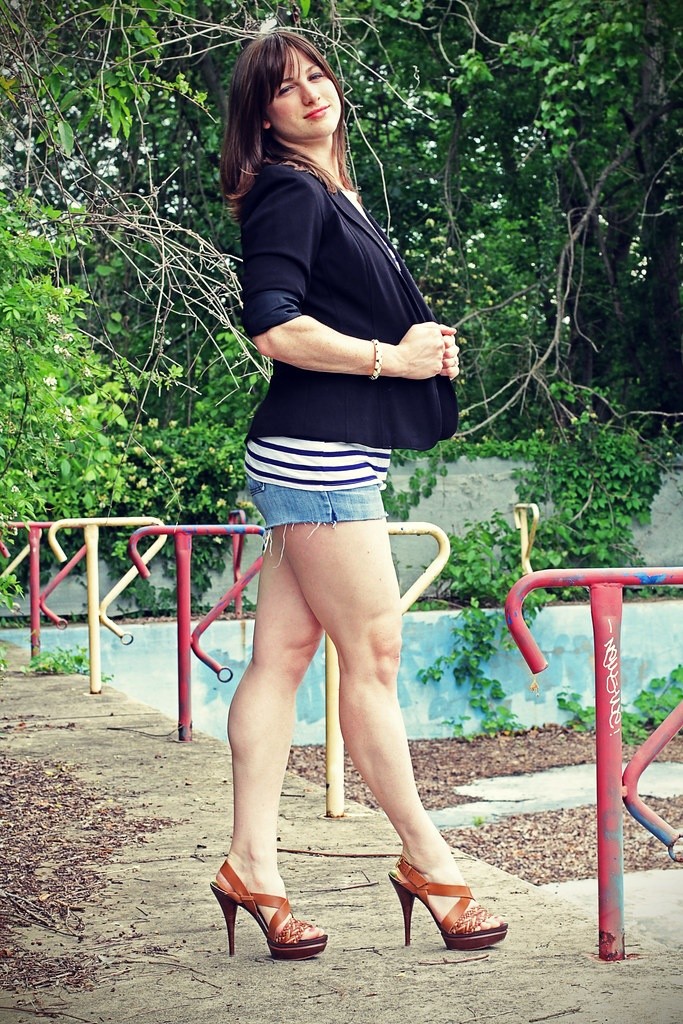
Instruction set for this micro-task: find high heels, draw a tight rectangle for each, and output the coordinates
[388,858,508,950]
[209,859,328,962]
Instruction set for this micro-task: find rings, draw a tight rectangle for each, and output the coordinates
[454,356,459,366]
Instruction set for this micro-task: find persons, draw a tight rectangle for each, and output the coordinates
[208,33,510,961]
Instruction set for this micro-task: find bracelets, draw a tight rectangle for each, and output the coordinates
[369,339,383,380]
